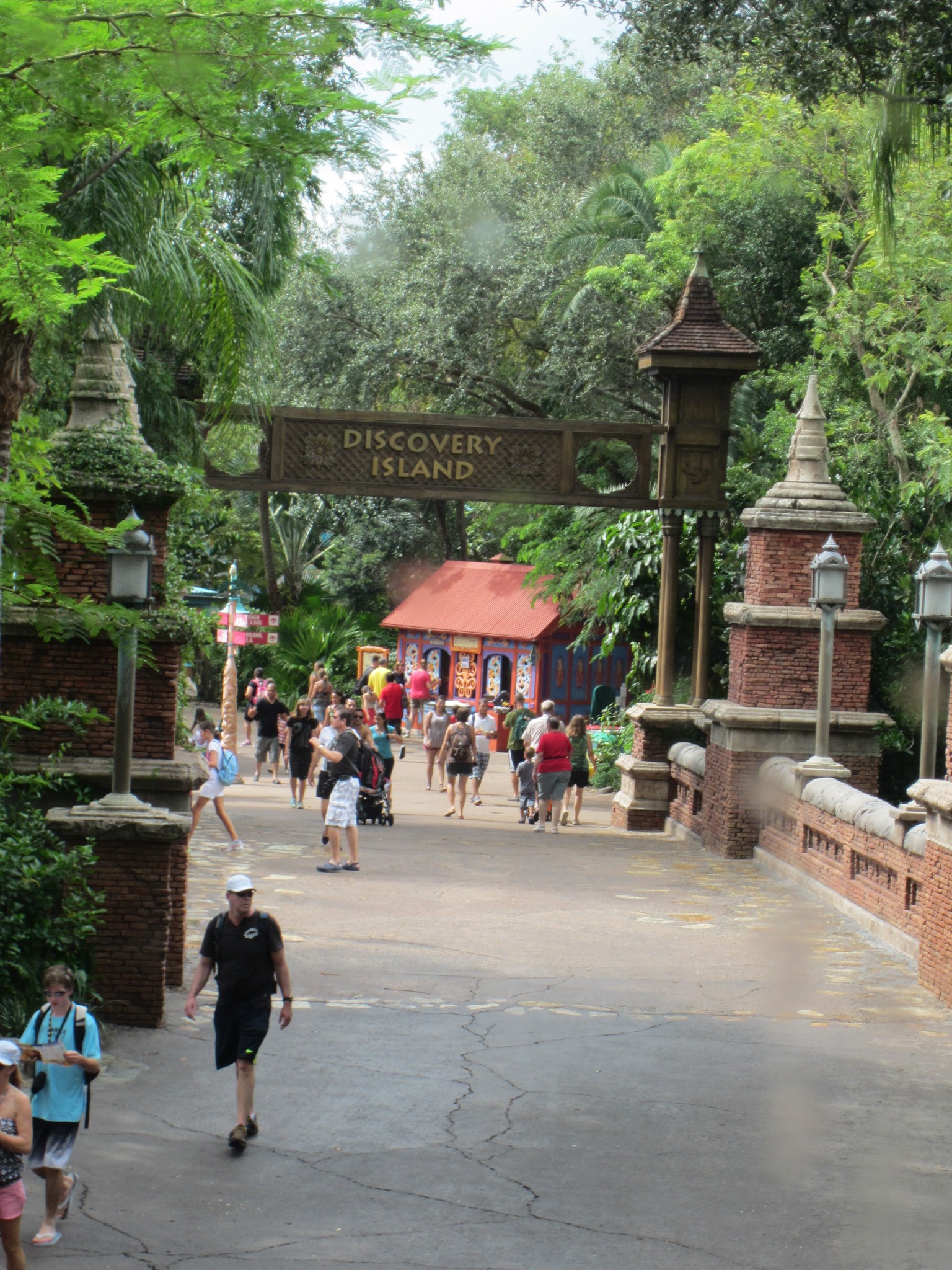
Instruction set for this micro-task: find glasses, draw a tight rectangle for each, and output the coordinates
[237,892,254,897]
[354,715,363,719]
[43,989,68,997]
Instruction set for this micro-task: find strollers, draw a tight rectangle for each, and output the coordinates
[356,749,394,826]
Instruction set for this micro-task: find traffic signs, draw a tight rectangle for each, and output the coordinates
[247,614,279,627]
[242,631,278,644]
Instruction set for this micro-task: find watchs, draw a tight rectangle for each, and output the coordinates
[282,995,293,1002]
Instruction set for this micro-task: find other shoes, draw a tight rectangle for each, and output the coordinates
[474,796,482,805]
[291,797,296,808]
[426,783,432,791]
[228,1124,248,1150]
[254,774,259,781]
[399,745,405,760]
[272,779,280,784]
[439,784,447,792]
[323,836,329,845]
[470,795,474,802]
[405,732,410,737]
[221,841,243,851]
[298,802,304,809]
[247,1116,259,1134]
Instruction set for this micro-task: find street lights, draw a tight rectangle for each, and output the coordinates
[889,539,952,823]
[790,531,852,778]
[87,504,152,813]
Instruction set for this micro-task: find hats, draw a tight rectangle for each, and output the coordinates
[0,1040,21,1067]
[227,875,256,893]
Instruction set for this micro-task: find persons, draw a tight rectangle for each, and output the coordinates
[188,651,597,871]
[185,874,292,1153]
[0,963,102,1270]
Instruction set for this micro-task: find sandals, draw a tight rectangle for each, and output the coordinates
[341,862,360,871]
[317,862,340,872]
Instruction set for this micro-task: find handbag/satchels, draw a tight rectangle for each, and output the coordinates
[353,675,367,696]
[400,684,409,709]
[218,749,238,786]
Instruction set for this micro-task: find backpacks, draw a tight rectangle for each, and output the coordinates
[252,679,267,705]
[334,730,384,789]
[449,724,470,760]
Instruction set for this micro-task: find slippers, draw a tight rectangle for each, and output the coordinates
[57,1172,78,1221]
[33,1233,63,1247]
[444,810,456,817]
[458,815,464,819]
